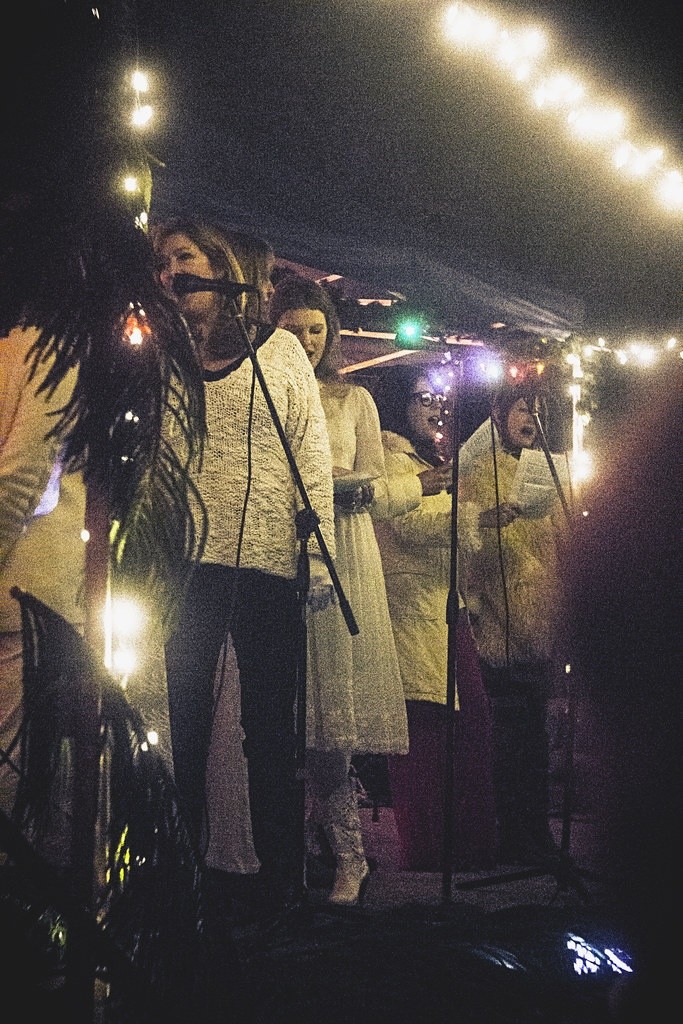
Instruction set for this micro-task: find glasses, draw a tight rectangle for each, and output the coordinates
[410,390,445,408]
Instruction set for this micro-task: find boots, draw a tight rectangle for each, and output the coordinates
[303,780,370,911]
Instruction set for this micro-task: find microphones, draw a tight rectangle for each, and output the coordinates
[171,272,258,296]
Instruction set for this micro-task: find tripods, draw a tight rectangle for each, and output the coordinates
[457,387,604,909]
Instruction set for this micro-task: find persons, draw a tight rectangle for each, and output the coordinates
[130,218,338,885]
[363,358,524,868]
[264,265,410,904]
[0,103,152,846]
[445,380,573,871]
